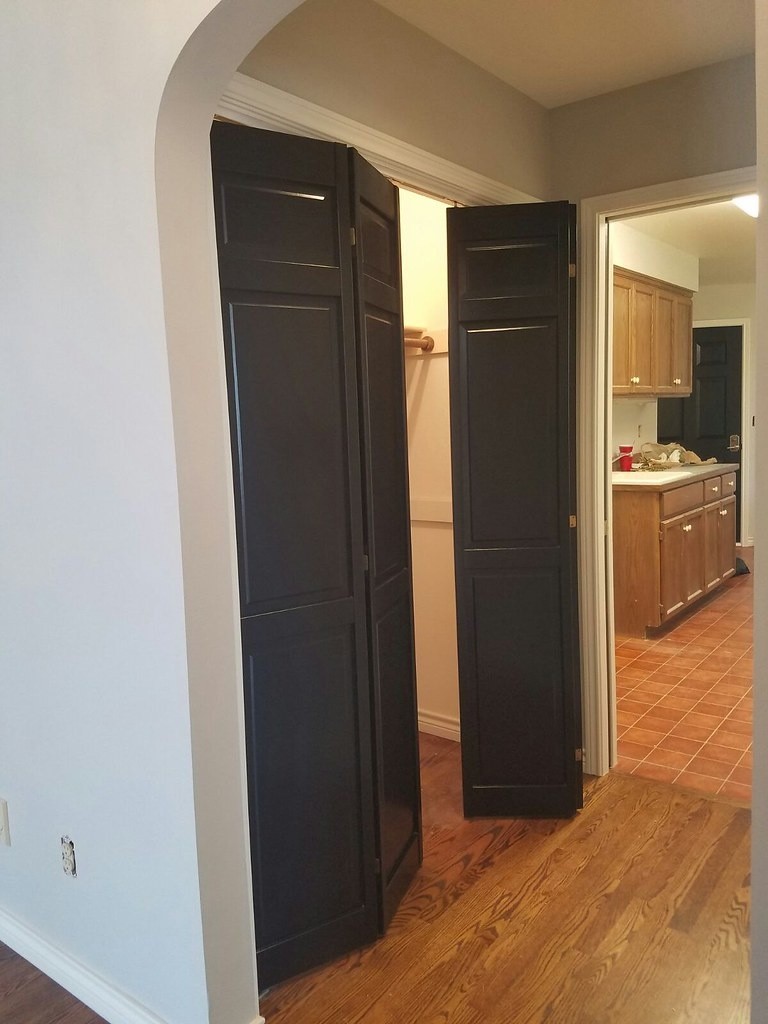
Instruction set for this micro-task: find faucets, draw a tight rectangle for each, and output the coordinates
[612,452,633,464]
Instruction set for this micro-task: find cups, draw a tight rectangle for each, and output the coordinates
[619,445,633,471]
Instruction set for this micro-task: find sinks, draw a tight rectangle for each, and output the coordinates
[612,469,693,486]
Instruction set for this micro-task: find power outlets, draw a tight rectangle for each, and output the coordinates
[637,425,641,438]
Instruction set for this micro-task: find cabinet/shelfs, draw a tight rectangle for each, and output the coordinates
[657,279,693,399]
[613,265,657,400]
[611,473,705,642]
[703,465,740,594]
